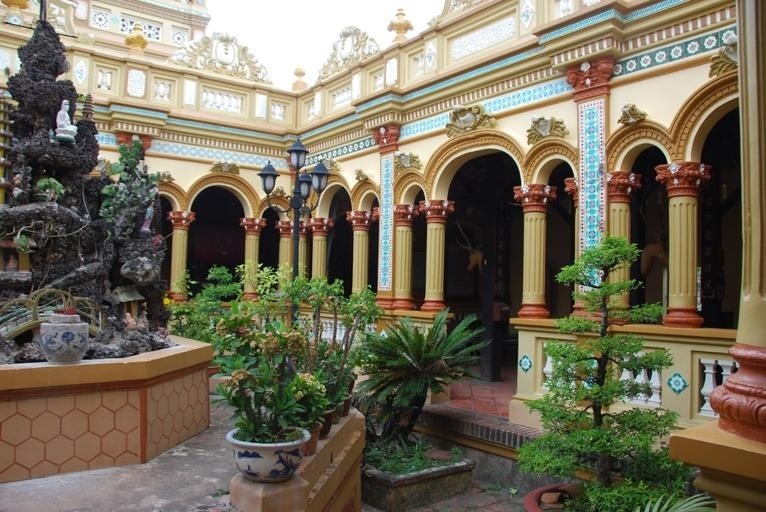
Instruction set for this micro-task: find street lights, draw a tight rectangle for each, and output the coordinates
[257,136,332,331]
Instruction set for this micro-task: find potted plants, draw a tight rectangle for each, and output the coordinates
[358,310,494,508]
[291,370,326,456]
[211,299,311,482]
[331,274,356,427]
[306,360,337,438]
[40,288,89,365]
[520,220,702,512]
